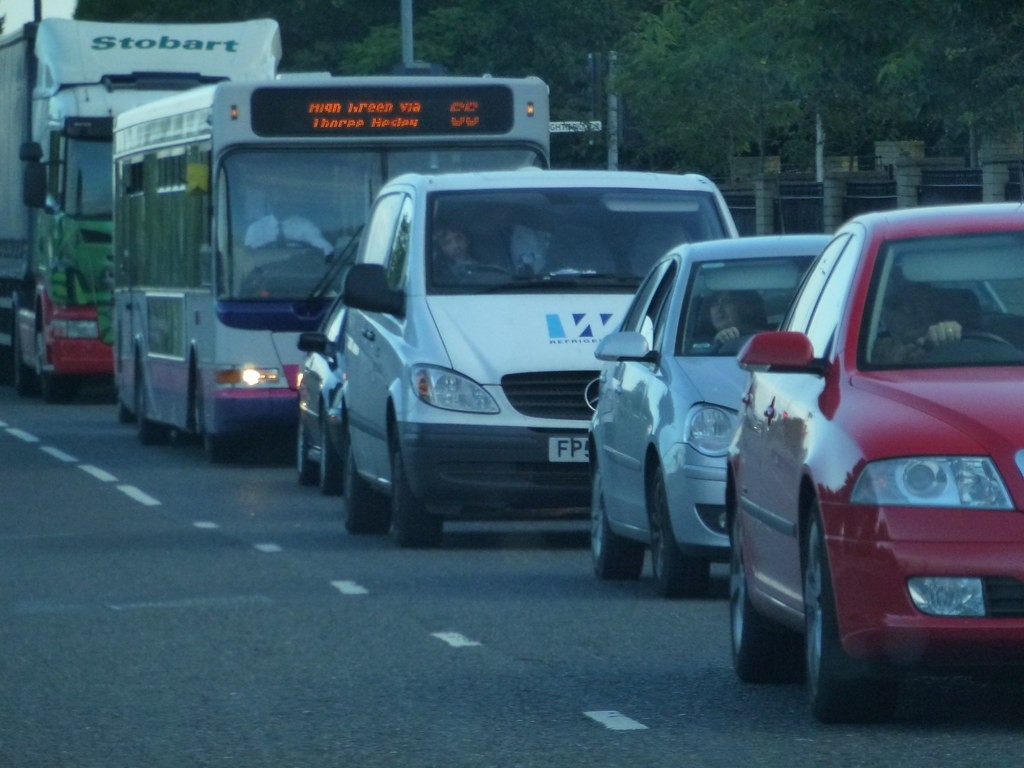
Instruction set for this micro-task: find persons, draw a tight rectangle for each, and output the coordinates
[322,151,372,213]
[245,186,335,262]
[869,278,983,366]
[438,224,511,288]
[690,291,767,356]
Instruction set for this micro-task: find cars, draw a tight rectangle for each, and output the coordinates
[587,235,831,597]
[297,292,346,494]
[725,201,1024,722]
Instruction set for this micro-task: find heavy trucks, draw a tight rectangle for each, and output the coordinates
[0,18,285,401]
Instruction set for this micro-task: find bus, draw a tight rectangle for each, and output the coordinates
[112,75,555,461]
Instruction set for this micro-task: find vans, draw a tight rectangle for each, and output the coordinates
[340,168,740,544]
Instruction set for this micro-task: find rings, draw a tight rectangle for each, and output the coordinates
[946,328,954,333]
